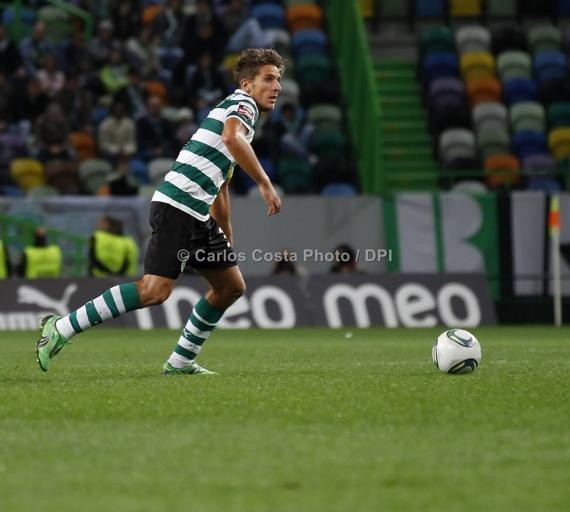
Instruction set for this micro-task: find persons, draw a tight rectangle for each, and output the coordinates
[327,243,356,273]
[87,214,141,279]
[35,45,287,376]
[272,246,294,274]
[0,236,13,279]
[15,227,64,279]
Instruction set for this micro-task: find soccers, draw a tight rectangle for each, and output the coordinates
[431,328,482,374]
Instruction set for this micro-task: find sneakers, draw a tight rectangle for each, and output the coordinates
[33,312,71,374]
[158,358,220,376]
